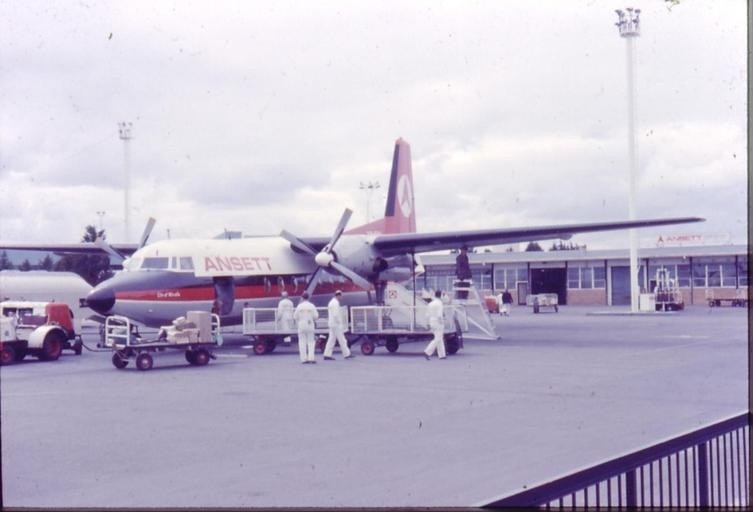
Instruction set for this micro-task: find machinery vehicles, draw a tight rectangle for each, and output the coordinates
[651,264,686,310]
[0,302,82,365]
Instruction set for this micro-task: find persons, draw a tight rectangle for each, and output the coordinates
[494,291,504,316]
[421,289,447,361]
[453,247,473,299]
[371,256,389,305]
[501,289,513,316]
[322,290,354,360]
[291,292,319,365]
[273,290,294,344]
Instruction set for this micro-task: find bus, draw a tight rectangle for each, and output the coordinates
[0,138,706,327]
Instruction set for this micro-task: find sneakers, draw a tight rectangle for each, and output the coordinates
[303,355,355,364]
[423,352,448,360]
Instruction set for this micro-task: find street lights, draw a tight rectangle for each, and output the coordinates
[614,4,642,312]
[118,119,135,244]
